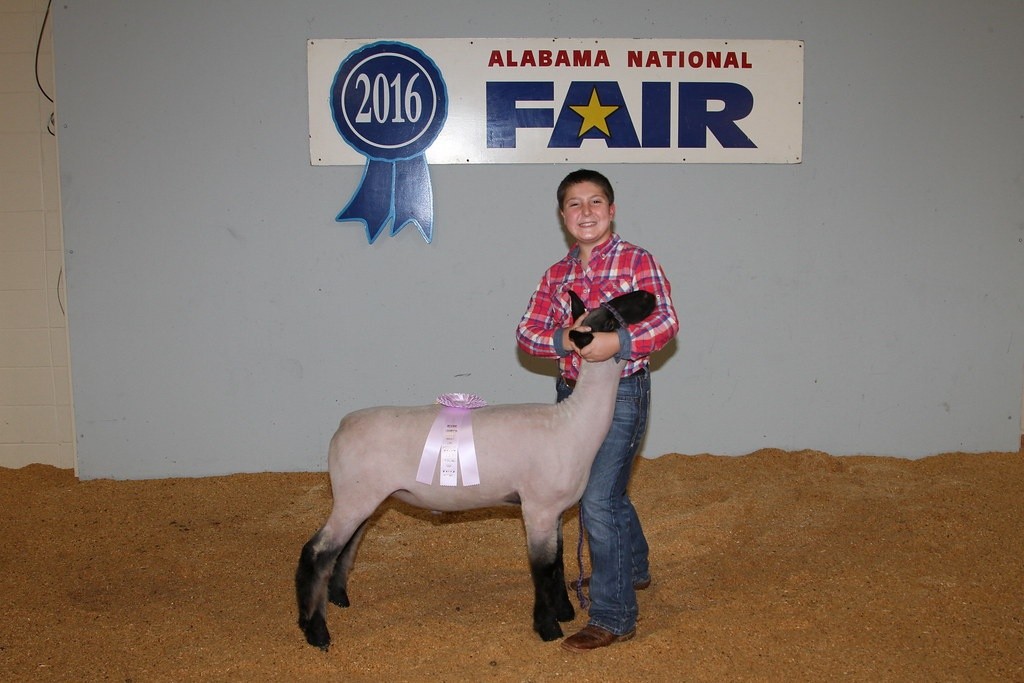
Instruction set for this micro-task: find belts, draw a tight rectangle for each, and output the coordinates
[562,368,645,389]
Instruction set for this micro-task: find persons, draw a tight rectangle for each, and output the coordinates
[517,169,679,649]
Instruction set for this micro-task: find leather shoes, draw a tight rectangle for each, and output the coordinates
[568,573,651,592]
[561,623,637,652]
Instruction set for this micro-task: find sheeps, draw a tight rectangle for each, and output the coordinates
[297,289,661,648]
